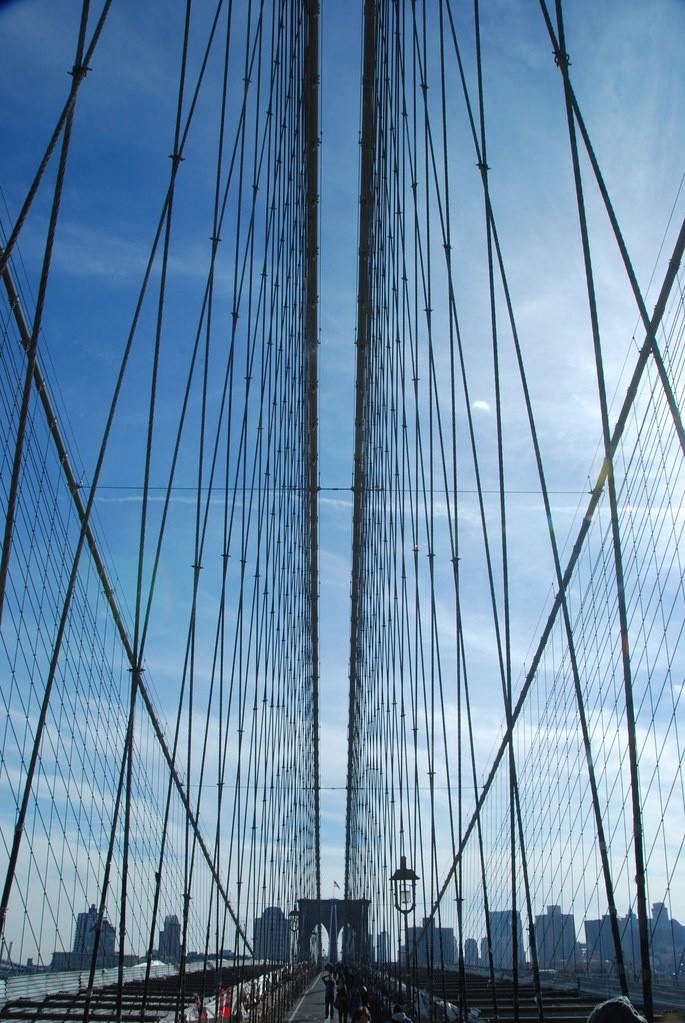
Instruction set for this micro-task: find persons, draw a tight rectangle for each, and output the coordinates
[322,960,412,1023]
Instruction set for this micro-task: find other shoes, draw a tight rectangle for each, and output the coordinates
[325,1016,328,1018]
[330,1017,334,1019]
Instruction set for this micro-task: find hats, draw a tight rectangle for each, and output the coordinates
[393,1005,405,1013]
